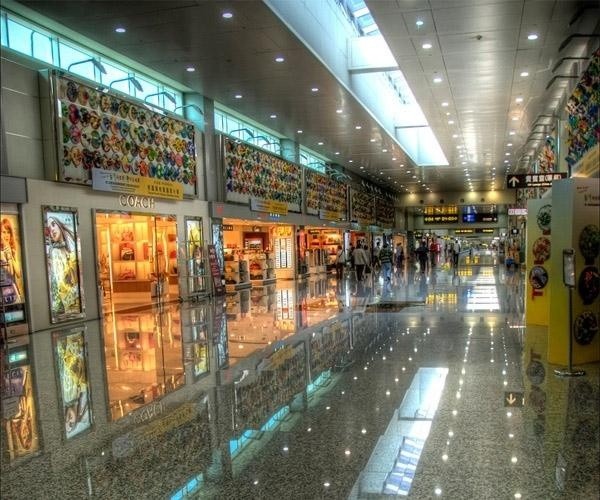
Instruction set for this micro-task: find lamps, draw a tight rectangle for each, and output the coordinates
[67,59,354,183]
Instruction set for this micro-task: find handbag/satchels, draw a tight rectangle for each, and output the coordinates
[364,264,371,274]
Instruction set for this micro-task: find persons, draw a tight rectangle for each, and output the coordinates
[9,371,35,451]
[450,240,461,267]
[347,237,404,280]
[414,241,431,274]
[335,245,346,280]
[1,218,23,305]
[46,215,81,314]
[51,334,92,436]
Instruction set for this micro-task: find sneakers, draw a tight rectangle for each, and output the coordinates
[384,277,390,281]
